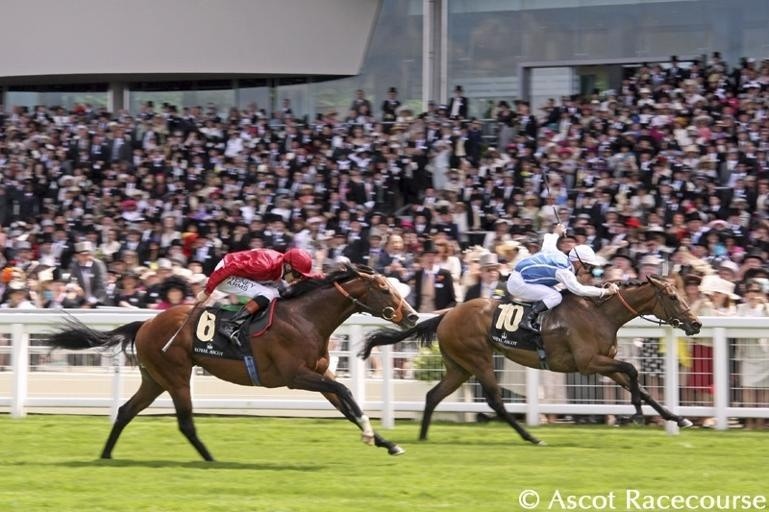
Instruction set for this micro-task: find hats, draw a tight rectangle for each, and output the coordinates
[453,85,462,92]
[420,240,439,255]
[74,242,93,255]
[608,248,635,266]
[480,254,501,268]
[684,212,763,299]
[386,87,396,92]
[711,52,721,57]
[669,56,677,62]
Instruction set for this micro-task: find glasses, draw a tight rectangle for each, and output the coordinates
[288,248,301,279]
[573,247,590,270]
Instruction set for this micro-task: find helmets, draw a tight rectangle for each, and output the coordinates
[282,247,311,273]
[568,246,599,266]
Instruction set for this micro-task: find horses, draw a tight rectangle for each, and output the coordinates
[352,273,702,448]
[38,260,419,463]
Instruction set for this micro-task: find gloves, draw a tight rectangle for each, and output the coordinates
[604,283,618,294]
[553,224,565,235]
[196,291,209,302]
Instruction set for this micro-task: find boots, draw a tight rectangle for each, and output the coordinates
[218,308,250,346]
[518,300,548,334]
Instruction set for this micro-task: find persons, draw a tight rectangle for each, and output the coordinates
[0,50,768,432]
